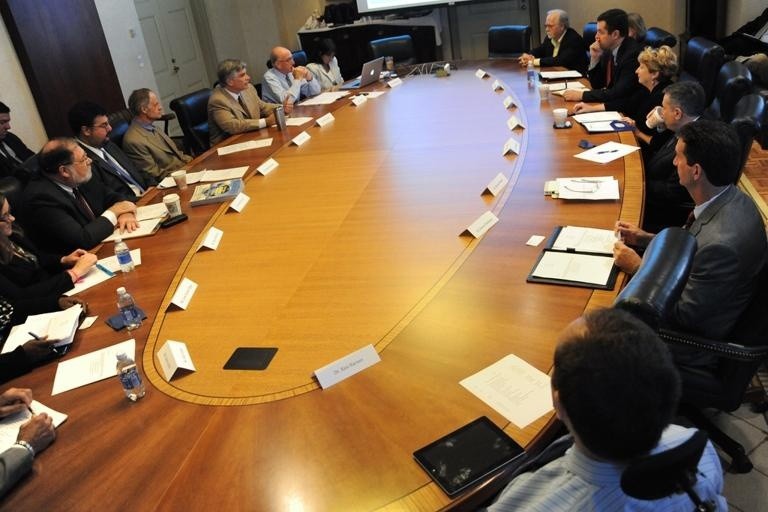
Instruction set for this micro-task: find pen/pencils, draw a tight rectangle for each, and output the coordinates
[23,400,35,415]
[618,230,622,241]
[598,150,617,154]
[96,264,113,276]
[28,332,58,354]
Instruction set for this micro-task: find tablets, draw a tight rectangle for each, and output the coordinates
[413,416,527,499]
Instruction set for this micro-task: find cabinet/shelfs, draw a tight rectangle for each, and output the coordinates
[297,6,450,80]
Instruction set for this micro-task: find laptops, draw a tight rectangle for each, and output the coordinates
[338,56,384,90]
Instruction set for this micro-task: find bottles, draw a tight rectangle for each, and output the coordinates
[527,61,535,83]
[116,353,145,402]
[272,105,286,130]
[114,237,136,276]
[117,287,145,340]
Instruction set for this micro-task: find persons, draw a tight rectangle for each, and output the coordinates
[0,388,56,502]
[518,7,729,233]
[483,307,730,512]
[0,295,87,385]
[2,37,343,318]
[613,120,768,341]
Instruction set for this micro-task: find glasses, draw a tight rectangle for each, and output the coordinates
[87,123,107,128]
[564,180,600,193]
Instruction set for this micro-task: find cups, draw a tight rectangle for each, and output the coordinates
[163,194,182,217]
[538,84,548,100]
[171,170,187,190]
[552,109,568,128]
[385,56,393,70]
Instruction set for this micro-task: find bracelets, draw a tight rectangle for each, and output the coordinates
[14,440,35,459]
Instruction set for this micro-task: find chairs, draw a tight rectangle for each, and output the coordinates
[488,25,532,58]
[584,22,598,59]
[645,27,677,49]
[106,108,191,169]
[368,35,416,68]
[655,327,768,473]
[169,88,212,155]
[705,61,753,123]
[729,94,766,185]
[683,36,725,107]
[616,228,699,330]
[267,50,308,68]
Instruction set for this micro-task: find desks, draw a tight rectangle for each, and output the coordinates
[0,58,647,512]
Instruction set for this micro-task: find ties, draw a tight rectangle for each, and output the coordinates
[73,187,94,218]
[688,211,695,228]
[0,144,21,165]
[238,96,252,119]
[607,59,611,89]
[286,74,292,87]
[102,150,144,193]
[515,436,574,474]
